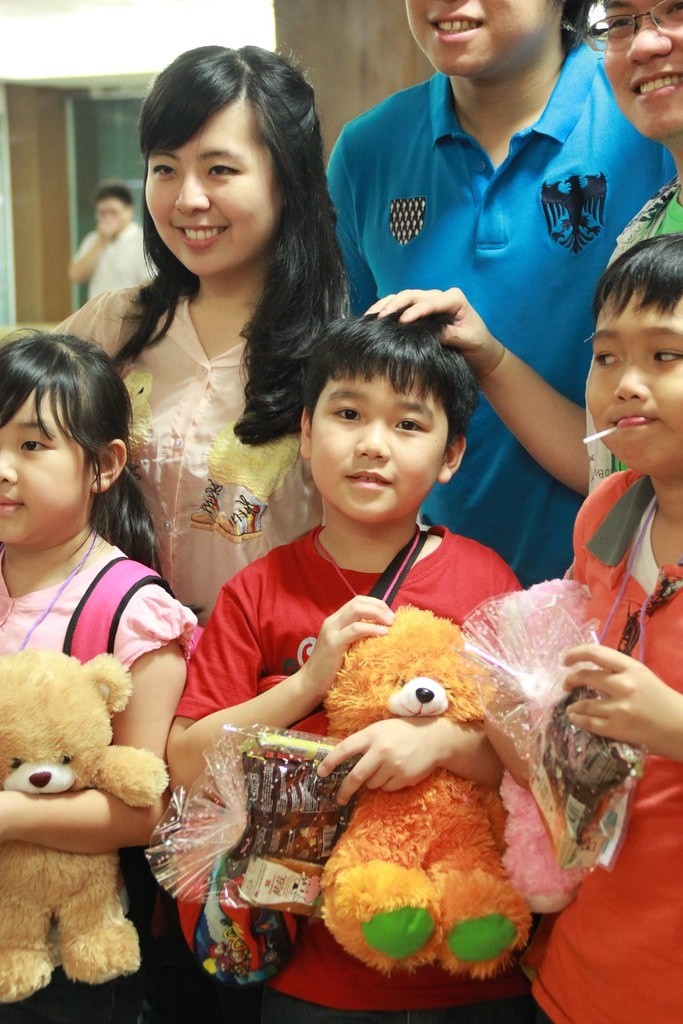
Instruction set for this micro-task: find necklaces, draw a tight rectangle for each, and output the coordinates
[19,532,98,651]
[596,499,683,665]
[316,523,421,602]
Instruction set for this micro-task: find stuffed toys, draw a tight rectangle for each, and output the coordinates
[0,649,170,1002]
[323,606,531,978]
[499,579,627,914]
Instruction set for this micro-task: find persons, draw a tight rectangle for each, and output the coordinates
[325,0,678,589]
[362,0,683,493]
[527,234,683,1024]
[167,310,530,1024]
[56,45,347,1024]
[68,185,159,301]
[0,327,197,1024]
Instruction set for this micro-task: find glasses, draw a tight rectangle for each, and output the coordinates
[584,0,683,54]
